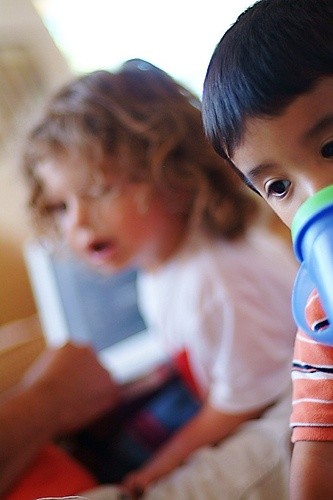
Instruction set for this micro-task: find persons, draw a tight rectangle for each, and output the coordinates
[20,58,301,500]
[200,0,333,500]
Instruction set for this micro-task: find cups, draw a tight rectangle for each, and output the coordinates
[290,185,333,346]
